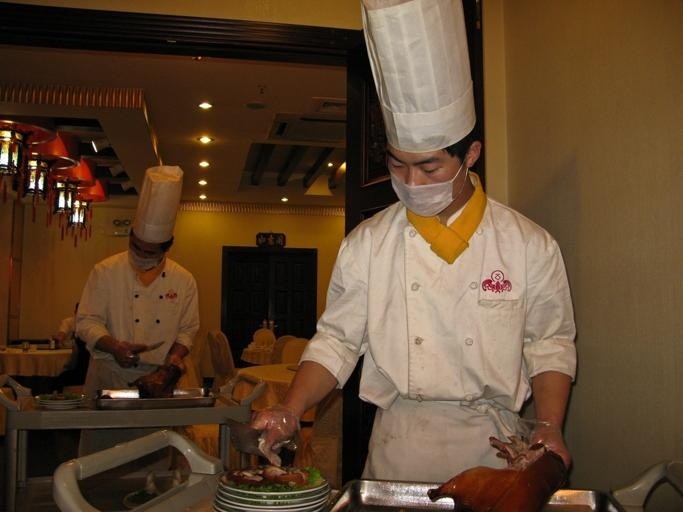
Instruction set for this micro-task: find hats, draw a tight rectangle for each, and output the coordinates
[133,165,184,244]
[359,1,478,154]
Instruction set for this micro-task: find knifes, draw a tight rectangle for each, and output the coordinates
[132,340,166,356]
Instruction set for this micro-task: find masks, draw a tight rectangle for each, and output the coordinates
[128,246,163,272]
[389,158,468,218]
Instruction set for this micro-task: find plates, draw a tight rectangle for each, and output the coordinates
[34,393,86,412]
[212,466,331,512]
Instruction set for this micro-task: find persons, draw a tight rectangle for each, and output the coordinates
[56,302,79,343]
[250,0,577,483]
[46,331,91,394]
[75,165,199,470]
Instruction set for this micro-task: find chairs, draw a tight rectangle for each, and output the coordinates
[164,328,308,470]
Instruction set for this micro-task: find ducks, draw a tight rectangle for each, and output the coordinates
[427,435,568,512]
[127,364,182,399]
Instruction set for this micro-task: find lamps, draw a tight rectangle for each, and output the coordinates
[0,118,105,249]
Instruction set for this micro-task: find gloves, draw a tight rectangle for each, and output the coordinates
[249,403,306,468]
[517,416,572,473]
